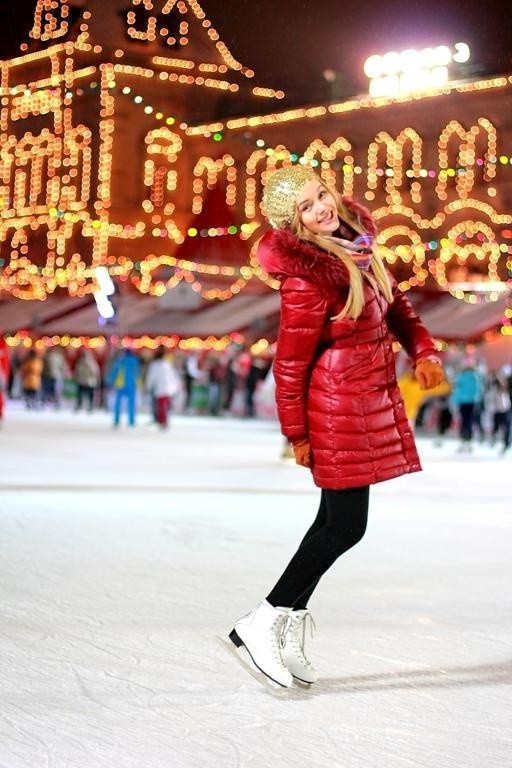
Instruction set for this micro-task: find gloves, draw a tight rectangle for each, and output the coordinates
[414,356,444,389]
[294,442,312,467]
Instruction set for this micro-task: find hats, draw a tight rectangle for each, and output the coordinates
[262,164,315,231]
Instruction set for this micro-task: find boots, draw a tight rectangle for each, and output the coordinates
[228,598,317,687]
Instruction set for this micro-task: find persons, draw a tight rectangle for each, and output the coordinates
[395,348,512,455]
[230,163,444,689]
[0,340,264,429]
